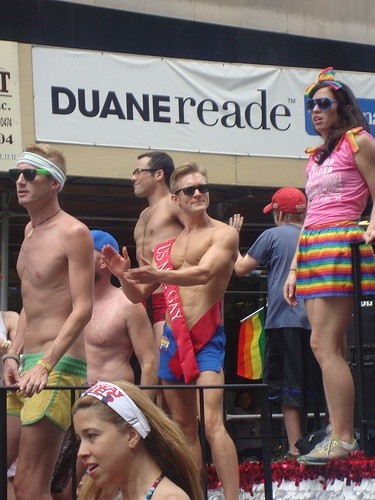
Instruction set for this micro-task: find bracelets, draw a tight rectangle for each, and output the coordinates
[36,360,52,374]
[2,354,20,366]
[288,268,296,272]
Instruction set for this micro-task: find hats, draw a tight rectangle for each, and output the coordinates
[90,230,120,256]
[262,187,306,214]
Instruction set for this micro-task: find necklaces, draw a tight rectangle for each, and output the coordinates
[27,208,62,238]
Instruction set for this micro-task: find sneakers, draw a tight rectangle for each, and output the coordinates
[296,434,360,466]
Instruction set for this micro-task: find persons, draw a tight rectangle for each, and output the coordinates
[70,380,190,500]
[102,163,240,500]
[59,230,160,500]
[284,67,375,466]
[133,153,186,419]
[0,311,20,500]
[229,187,312,464]
[1,146,94,500]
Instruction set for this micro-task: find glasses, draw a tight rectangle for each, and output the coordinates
[8,168,51,182]
[133,167,155,176]
[174,184,210,196]
[305,97,337,114]
